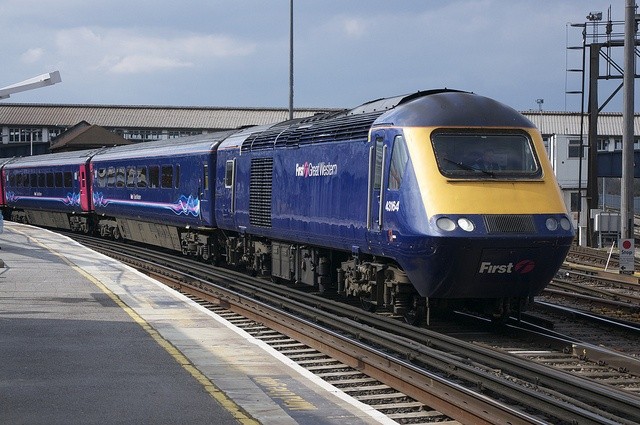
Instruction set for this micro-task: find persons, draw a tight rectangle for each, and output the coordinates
[471,149,500,172]
[436,150,456,170]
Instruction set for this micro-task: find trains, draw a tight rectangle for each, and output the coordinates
[0,88,575,325]
